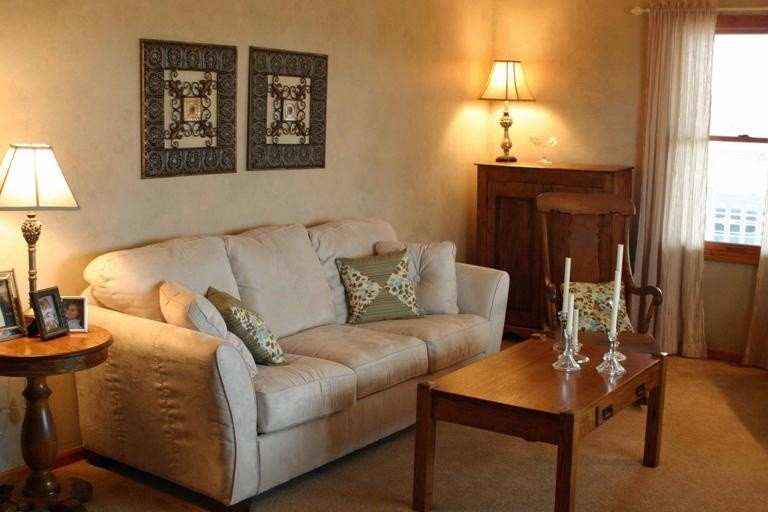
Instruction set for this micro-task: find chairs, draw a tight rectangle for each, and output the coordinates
[536,193,663,404]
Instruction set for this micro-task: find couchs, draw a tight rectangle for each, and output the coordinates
[75,216,511,511]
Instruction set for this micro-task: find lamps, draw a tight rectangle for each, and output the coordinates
[0,143,81,308]
[477,59,537,162]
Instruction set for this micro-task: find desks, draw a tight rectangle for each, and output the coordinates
[0,321,114,511]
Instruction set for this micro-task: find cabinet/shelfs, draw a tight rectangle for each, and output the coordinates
[475,161,634,338]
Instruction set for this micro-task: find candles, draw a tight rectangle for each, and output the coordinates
[561,244,624,346]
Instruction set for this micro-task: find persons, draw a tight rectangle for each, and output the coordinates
[63,301,83,329]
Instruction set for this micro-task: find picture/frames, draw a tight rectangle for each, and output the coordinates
[139,38,238,179]
[0,269,88,342]
[246,46,328,171]
